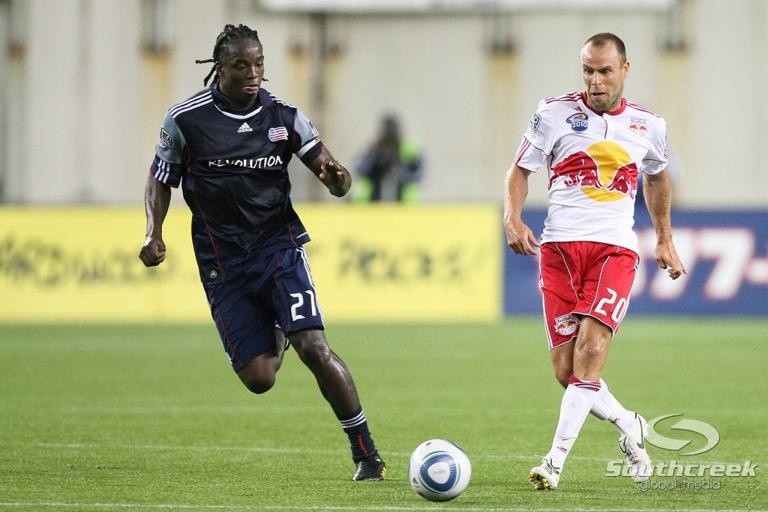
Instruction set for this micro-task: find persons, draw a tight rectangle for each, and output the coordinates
[136,27,387,482]
[355,116,419,204]
[502,32,686,492]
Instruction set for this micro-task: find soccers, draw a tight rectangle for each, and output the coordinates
[409,439,471,501]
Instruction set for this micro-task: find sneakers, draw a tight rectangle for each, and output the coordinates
[618,410,654,483]
[275,320,290,351]
[352,449,387,482]
[529,453,562,491]
